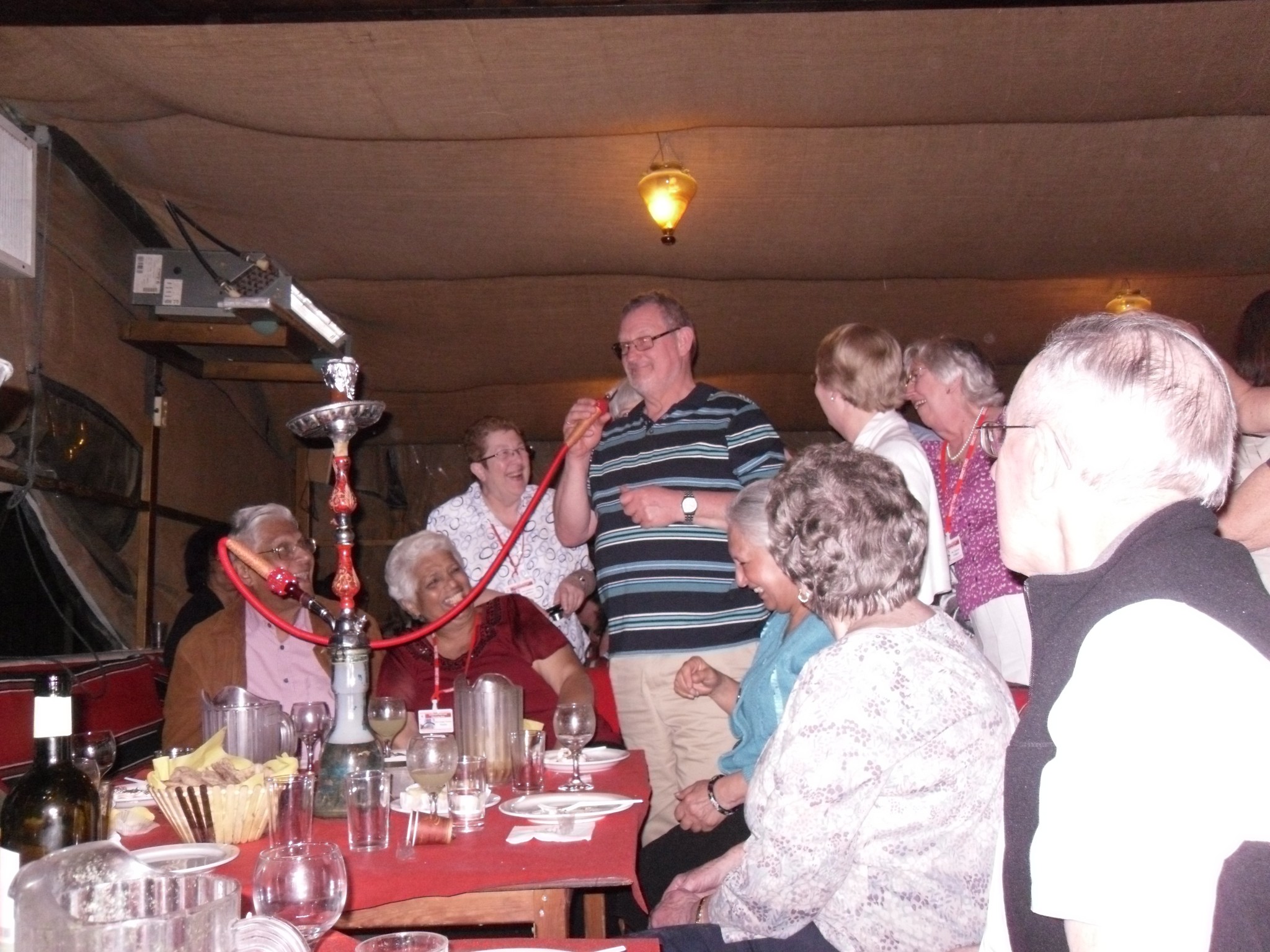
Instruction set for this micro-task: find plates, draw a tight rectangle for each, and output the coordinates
[499,791,634,823]
[390,792,503,817]
[544,747,632,774]
[131,843,240,876]
[114,783,156,808]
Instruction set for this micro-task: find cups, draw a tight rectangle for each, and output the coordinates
[345,770,393,852]
[253,842,348,952]
[70,729,117,839]
[406,811,452,848]
[508,729,545,794]
[264,774,314,849]
[354,931,449,952]
[149,622,167,649]
[447,756,486,833]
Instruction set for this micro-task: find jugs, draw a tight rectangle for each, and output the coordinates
[199,685,298,765]
[8,840,312,952]
[453,671,524,787]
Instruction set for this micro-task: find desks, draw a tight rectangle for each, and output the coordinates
[101,752,653,941]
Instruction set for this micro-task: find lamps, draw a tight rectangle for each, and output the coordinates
[1106,278,1154,314]
[635,131,698,247]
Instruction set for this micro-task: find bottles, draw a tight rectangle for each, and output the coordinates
[0,671,102,868]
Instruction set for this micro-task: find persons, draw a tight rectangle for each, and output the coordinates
[553,291,787,846]
[164,523,239,669]
[427,416,597,661]
[902,336,1033,685]
[1176,289,1270,590]
[162,504,387,755]
[634,480,837,913]
[978,312,1270,952]
[627,441,1021,952]
[814,323,951,605]
[376,530,620,750]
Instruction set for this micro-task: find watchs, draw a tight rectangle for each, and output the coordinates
[682,490,697,523]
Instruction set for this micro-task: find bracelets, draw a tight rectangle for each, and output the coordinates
[579,576,585,581]
[707,774,739,815]
[696,896,707,923]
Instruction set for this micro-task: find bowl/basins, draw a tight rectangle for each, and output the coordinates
[146,783,282,844]
[154,748,196,759]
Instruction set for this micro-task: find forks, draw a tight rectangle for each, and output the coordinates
[538,799,643,812]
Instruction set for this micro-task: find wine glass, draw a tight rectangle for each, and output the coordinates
[367,697,408,757]
[290,701,332,781]
[407,733,459,814]
[552,703,597,791]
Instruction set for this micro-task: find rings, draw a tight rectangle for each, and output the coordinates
[565,419,570,424]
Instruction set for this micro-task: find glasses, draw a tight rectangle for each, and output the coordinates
[975,421,1037,458]
[611,328,679,361]
[256,538,317,561]
[475,447,532,464]
[904,366,931,390]
